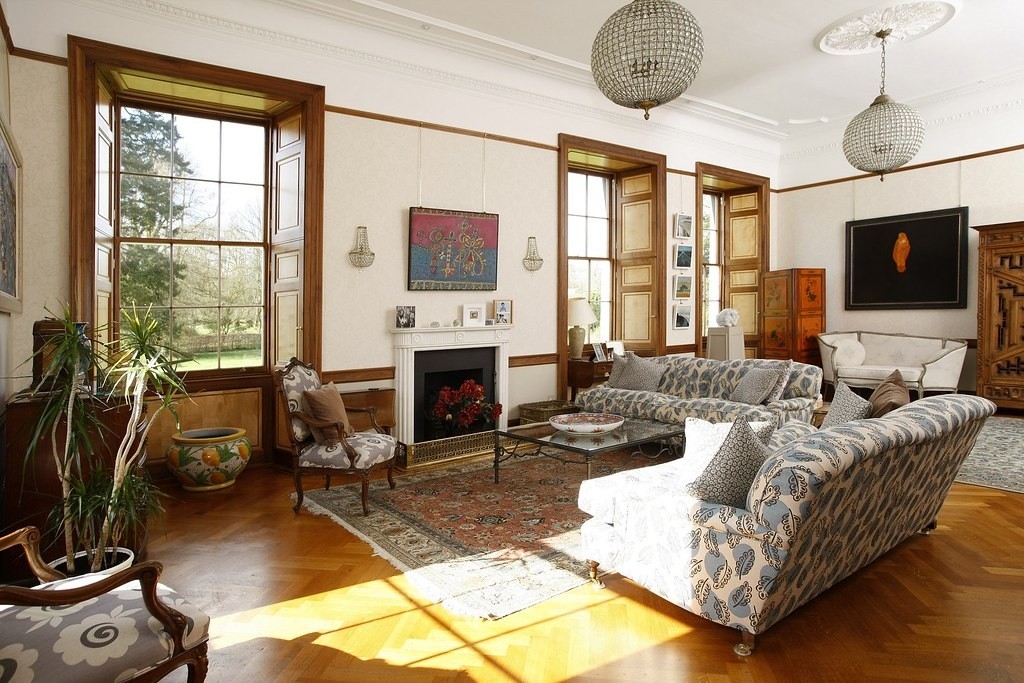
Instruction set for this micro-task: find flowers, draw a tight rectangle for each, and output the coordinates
[432,379,503,429]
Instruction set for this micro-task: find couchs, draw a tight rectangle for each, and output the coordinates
[579,393,996,656]
[576,356,823,444]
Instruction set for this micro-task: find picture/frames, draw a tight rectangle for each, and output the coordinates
[673,212,694,329]
[462,299,514,328]
[0,110,23,316]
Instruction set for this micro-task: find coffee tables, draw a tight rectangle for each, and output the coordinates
[493,415,685,485]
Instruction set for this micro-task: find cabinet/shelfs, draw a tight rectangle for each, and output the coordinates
[970,220,1024,408]
[1,389,149,583]
[760,268,827,368]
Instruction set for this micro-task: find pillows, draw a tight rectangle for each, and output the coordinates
[302,381,353,445]
[685,369,910,508]
[609,353,794,405]
[831,338,864,365]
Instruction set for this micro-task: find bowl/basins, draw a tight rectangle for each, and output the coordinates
[550,430,626,450]
[549,413,625,435]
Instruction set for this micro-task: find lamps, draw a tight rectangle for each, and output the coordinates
[349,226,543,270]
[844,206,968,310]
[591,0,924,179]
[568,298,597,359]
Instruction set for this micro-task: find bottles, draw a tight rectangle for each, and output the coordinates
[71,321,91,387]
[165,426,253,492]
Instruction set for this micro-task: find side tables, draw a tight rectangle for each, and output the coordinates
[568,358,613,401]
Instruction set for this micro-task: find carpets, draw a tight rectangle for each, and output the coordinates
[289,446,687,621]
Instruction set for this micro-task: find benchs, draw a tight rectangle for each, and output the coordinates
[816,330,967,399]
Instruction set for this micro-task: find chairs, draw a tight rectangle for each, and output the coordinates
[276,357,396,517]
[0,526,209,683]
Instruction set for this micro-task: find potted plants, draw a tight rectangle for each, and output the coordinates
[0,297,201,584]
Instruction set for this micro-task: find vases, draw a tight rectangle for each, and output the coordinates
[165,427,252,491]
